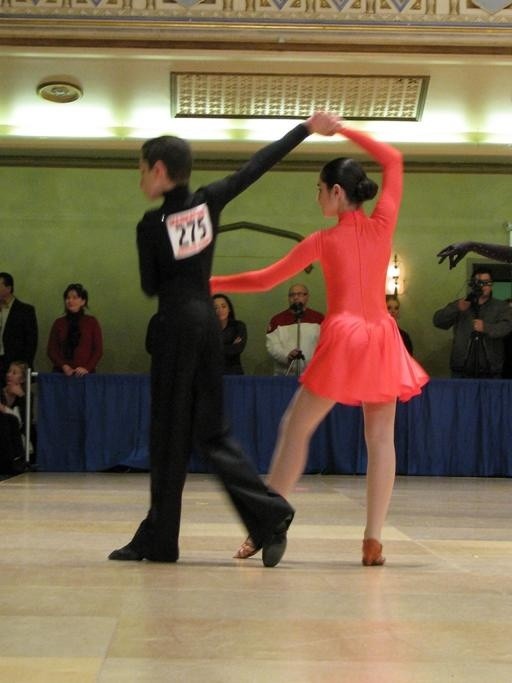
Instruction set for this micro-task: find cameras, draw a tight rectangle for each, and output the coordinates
[470,272,484,297]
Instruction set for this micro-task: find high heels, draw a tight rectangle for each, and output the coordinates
[363,539,385,565]
[233,535,260,558]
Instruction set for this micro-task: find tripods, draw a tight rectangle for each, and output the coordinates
[284,318,307,376]
[461,304,497,379]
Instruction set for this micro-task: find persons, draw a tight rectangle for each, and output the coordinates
[437,238,510,269]
[46,283,103,378]
[432,268,512,379]
[266,284,324,376]
[108,108,344,567]
[211,294,248,375]
[0,360,38,478]
[0,272,37,392]
[384,294,413,358]
[210,125,430,568]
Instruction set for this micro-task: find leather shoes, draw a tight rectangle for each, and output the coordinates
[263,514,291,566]
[109,544,143,560]
